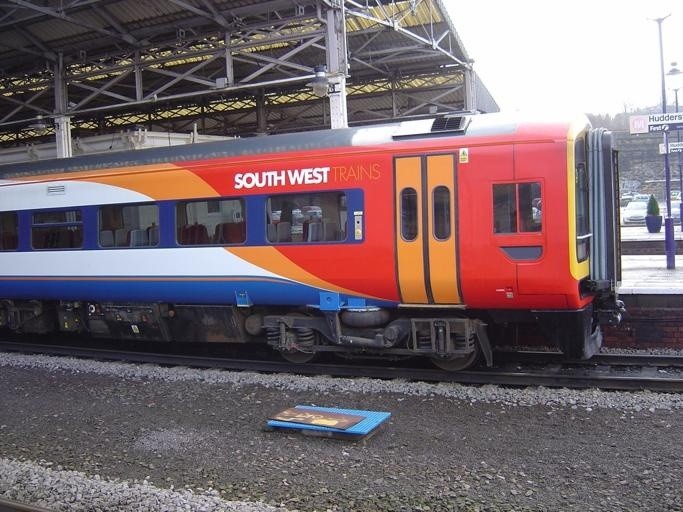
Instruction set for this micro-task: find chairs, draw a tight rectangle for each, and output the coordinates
[99,226,159,247]
[181,222,242,244]
[267,219,340,242]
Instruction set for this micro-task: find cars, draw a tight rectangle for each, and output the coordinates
[617,186,682,231]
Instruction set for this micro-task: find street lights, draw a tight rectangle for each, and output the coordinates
[643,0,676,270]
[665,61,683,233]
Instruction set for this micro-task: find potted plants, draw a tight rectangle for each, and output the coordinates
[644,194,661,233]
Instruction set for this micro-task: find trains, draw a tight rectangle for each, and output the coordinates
[0,105,628,369]
